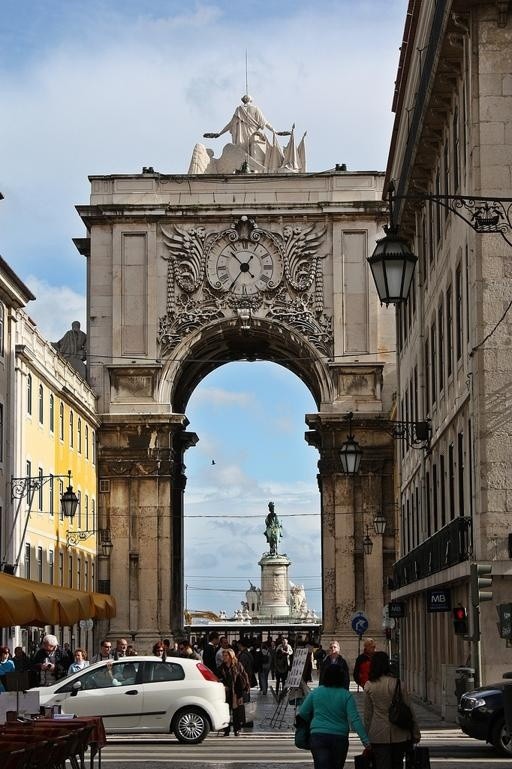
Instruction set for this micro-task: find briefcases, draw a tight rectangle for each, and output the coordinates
[238,705,245,722]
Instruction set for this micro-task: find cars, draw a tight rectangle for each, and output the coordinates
[457,678,512,755]
[24,656,231,744]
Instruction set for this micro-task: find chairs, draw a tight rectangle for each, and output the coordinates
[1,714,109,769]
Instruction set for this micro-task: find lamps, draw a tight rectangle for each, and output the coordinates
[361,524,400,555]
[372,501,398,535]
[363,176,511,309]
[335,411,432,479]
[65,528,114,557]
[9,468,83,517]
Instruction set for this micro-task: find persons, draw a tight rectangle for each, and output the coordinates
[203,94,292,174]
[352,637,375,692]
[149,629,328,736]
[296,664,373,769]
[0,634,138,692]
[48,320,88,364]
[318,641,350,691]
[363,650,422,768]
[263,502,284,544]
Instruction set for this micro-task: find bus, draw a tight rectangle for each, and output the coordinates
[184,624,323,670]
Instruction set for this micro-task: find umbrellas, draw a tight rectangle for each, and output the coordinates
[0,571,96,630]
[92,592,118,620]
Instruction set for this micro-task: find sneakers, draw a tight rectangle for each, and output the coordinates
[224,731,241,737]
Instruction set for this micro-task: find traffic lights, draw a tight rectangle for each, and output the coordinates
[469,563,493,602]
[453,606,467,635]
[495,603,512,638]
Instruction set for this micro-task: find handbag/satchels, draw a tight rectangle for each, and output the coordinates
[235,674,250,700]
[293,716,310,749]
[406,743,429,768]
[389,678,415,727]
